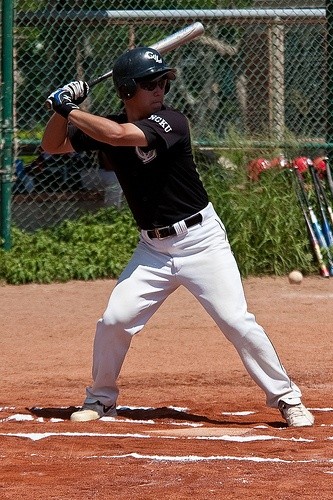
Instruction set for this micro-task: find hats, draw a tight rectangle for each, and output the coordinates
[137,73,175,84]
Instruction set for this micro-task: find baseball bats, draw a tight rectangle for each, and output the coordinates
[293,167,333,276]
[290,169,330,278]
[44,21,206,112]
[314,169,333,224]
[308,164,333,248]
[322,158,333,197]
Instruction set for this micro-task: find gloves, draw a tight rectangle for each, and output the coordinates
[50,89,79,119]
[63,80,89,105]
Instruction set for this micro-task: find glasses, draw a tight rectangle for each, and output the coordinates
[137,78,168,92]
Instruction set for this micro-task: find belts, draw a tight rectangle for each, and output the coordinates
[148,213,202,239]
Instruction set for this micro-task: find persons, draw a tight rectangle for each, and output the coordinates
[40,47,315,428]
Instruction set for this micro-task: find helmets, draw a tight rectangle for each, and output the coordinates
[112,49,178,99]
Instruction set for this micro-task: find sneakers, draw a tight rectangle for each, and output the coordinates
[69,401,118,422]
[277,399,315,426]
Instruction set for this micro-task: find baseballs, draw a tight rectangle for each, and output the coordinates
[288,270,303,284]
